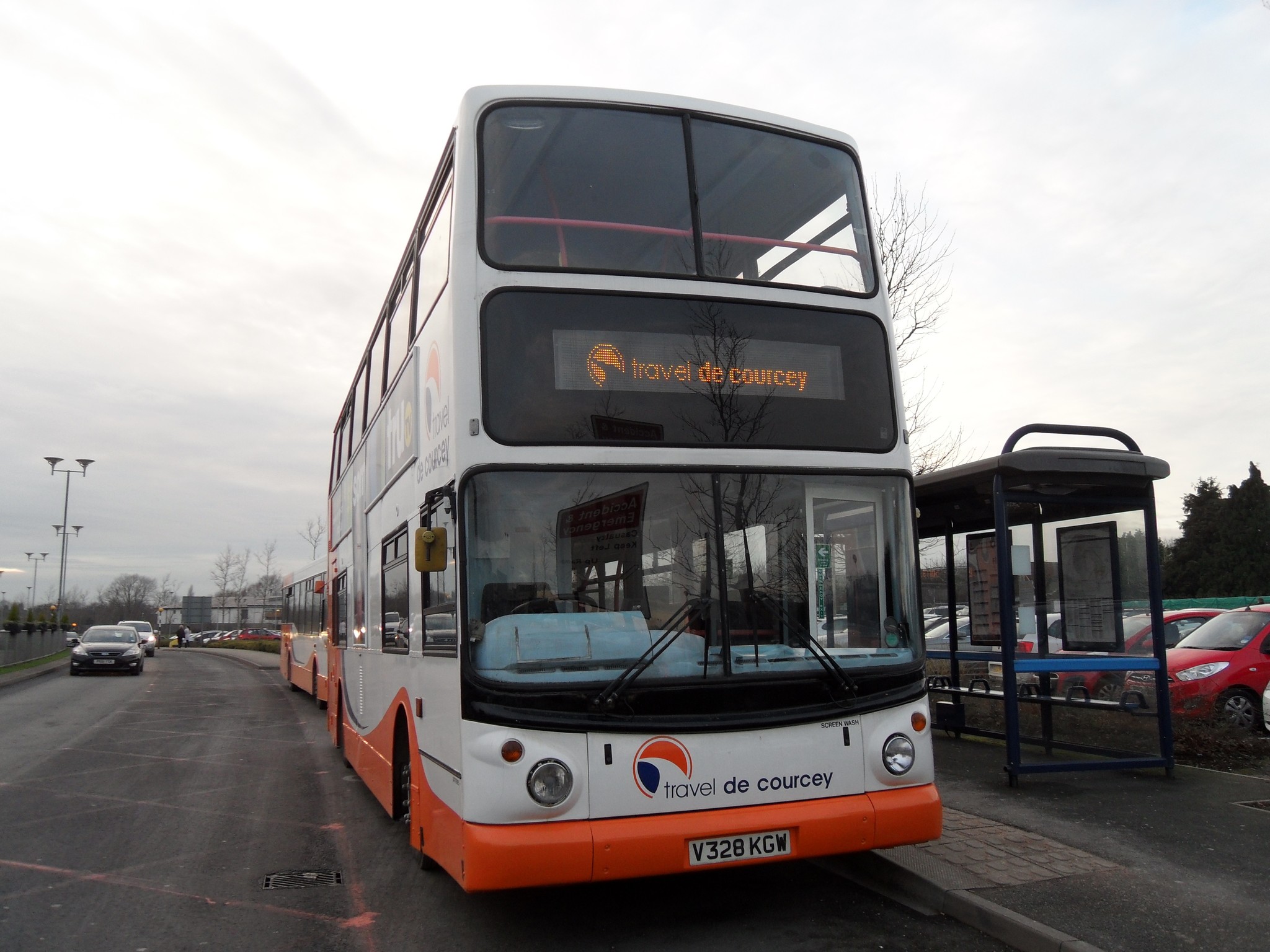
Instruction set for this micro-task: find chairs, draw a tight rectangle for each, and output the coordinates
[466,572,781,647]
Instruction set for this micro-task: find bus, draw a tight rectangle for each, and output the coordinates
[323,81,944,897]
[280,556,329,709]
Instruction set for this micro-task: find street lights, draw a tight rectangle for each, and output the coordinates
[157,608,164,649]
[44,456,95,630]
[25,553,49,615]
[26,586,32,613]
[49,605,56,623]
[1,591,6,601]
[51,524,85,626]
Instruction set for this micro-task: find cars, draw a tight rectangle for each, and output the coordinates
[117,620,159,657]
[818,599,1270,733]
[69,624,149,676]
[65,632,81,647]
[154,628,281,648]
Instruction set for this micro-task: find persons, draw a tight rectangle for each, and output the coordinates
[176,625,185,648]
[183,625,191,648]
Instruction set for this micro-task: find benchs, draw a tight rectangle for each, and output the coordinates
[927,673,1147,755]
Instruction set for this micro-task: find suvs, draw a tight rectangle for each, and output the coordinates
[385,611,455,653]
